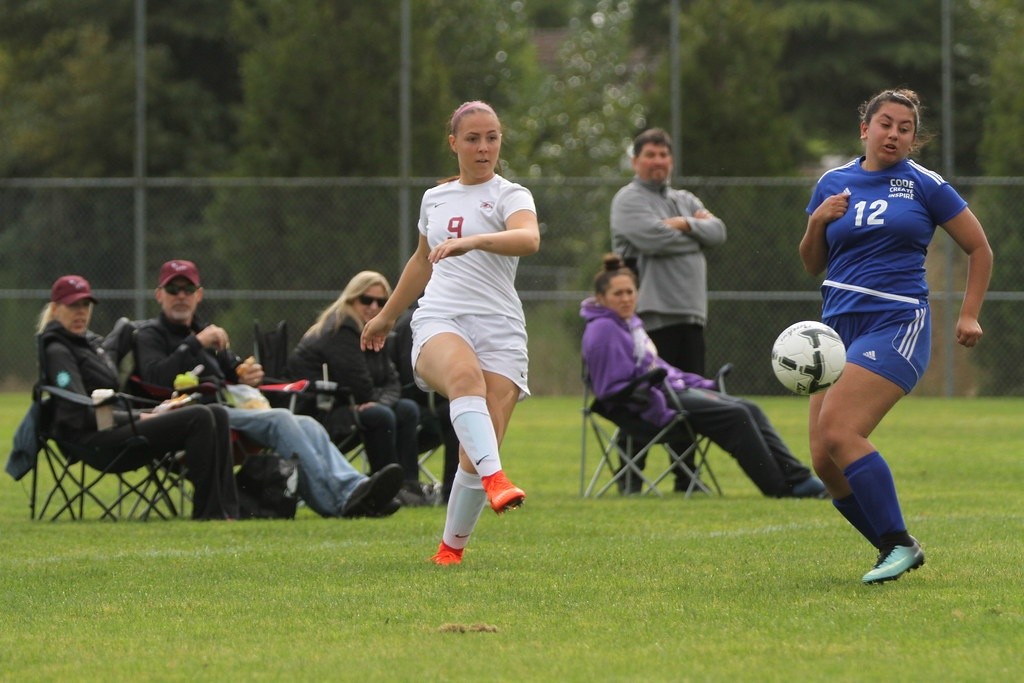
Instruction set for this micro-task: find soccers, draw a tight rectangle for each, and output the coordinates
[772,320,847,395]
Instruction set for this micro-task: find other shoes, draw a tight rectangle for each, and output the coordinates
[791,476,832,497]
[339,463,403,517]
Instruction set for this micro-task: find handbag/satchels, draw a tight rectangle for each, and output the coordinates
[235,447,300,520]
[252,319,290,383]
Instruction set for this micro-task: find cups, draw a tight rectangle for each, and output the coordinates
[315,380,338,410]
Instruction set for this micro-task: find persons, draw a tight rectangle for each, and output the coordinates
[360,100,540,566]
[37,275,238,522]
[800,87,993,583]
[609,128,726,495]
[275,270,429,507]
[579,253,829,499]
[392,288,459,507]
[135,260,404,521]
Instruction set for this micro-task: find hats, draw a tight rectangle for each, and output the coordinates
[51,275,99,306]
[158,259,200,287]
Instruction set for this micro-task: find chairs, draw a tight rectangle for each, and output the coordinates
[252,320,371,476]
[579,354,734,499]
[29,334,177,524]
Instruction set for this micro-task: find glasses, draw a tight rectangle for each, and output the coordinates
[358,294,387,306]
[163,281,200,294]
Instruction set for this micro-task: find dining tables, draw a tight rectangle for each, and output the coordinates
[115,320,311,519]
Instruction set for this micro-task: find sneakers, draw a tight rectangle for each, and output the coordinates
[482,470,524,513]
[862,536,924,584]
[431,543,461,566]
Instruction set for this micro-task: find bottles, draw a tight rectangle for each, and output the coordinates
[175,364,205,406]
